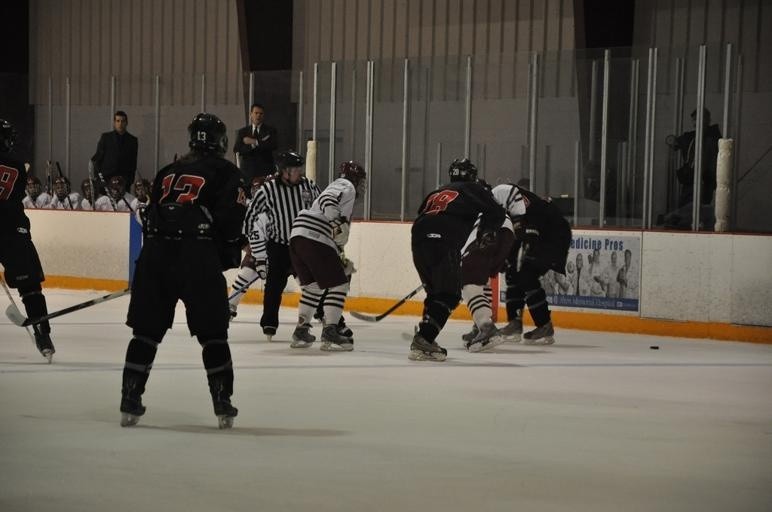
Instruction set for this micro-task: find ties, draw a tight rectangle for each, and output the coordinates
[118,135,124,163]
[253,125,259,138]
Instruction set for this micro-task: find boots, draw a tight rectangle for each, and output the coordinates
[460,322,506,353]
[522,321,556,346]
[290,322,317,349]
[32,327,55,364]
[497,319,524,344]
[120,383,147,427]
[260,313,280,343]
[209,383,239,431]
[319,323,355,352]
[407,333,448,362]
[335,316,353,337]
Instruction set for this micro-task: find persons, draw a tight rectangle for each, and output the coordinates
[673,107,722,205]
[120,113,242,430]
[541,247,634,297]
[245,150,352,341]
[229,175,279,322]
[289,161,366,352]
[462,211,517,352]
[410,159,509,361]
[0,110,156,361]
[233,103,278,201]
[473,179,572,346]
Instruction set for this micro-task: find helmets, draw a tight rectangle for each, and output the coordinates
[54,177,71,200]
[186,112,229,157]
[250,175,264,198]
[0,118,17,156]
[338,161,366,186]
[108,175,126,199]
[448,157,478,183]
[275,150,305,167]
[26,176,42,197]
[135,177,151,198]
[81,178,97,199]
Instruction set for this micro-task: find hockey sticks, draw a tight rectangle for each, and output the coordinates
[4,287,133,328]
[350,285,425,322]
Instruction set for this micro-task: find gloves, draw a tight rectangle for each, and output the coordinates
[329,216,350,246]
[221,236,243,270]
[517,252,537,277]
[477,232,500,257]
[511,214,526,242]
[255,257,270,279]
[340,257,353,277]
